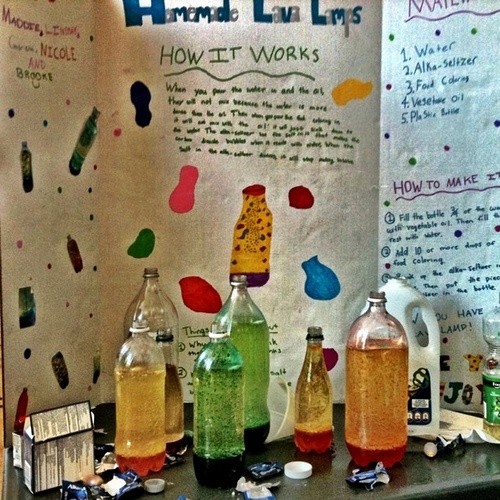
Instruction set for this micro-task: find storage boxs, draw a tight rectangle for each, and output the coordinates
[21,399,97,495]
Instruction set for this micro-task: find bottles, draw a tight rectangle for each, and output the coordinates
[113,268,186,479]
[295,326,334,454]
[345,290,407,467]
[194,276,270,488]
[483,292,500,439]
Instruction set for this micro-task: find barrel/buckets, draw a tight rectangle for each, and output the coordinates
[357,276,440,438]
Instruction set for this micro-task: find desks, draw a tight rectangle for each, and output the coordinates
[1,404,500,500]
